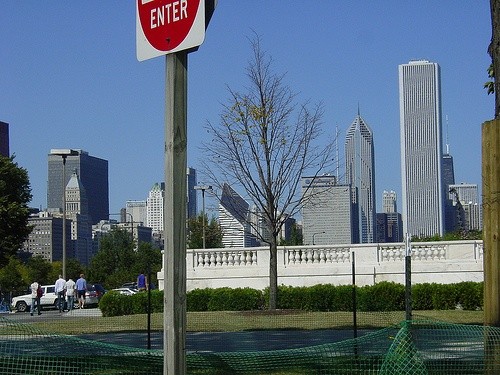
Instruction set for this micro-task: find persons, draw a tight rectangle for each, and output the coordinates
[75,274,88,310]
[63,276,76,312]
[0,292,6,312]
[6,291,13,313]
[54,274,67,312]
[137,272,149,292]
[29,280,45,316]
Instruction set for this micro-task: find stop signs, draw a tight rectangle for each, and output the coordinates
[136,0,205,62]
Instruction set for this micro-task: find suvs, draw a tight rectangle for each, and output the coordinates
[11,284,105,313]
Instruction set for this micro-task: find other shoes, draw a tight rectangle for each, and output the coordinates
[31,313,33,316]
[38,313,42,315]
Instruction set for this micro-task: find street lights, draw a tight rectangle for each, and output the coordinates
[50,148,79,281]
[194,186,212,249]
[312,232,326,245]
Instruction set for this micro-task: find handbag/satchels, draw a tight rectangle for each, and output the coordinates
[36,285,42,298]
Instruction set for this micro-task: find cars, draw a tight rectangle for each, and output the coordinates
[105,282,144,297]
